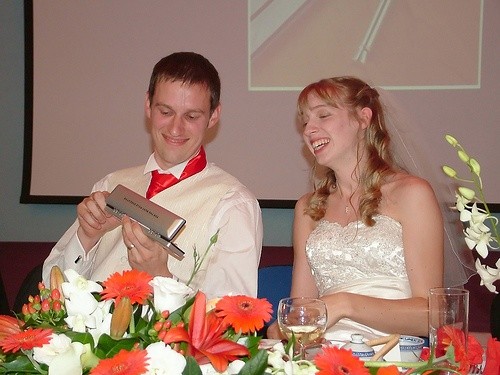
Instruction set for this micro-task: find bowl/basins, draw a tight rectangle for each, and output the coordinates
[398,334,424,369]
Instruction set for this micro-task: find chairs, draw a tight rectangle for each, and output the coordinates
[258,265,429,347]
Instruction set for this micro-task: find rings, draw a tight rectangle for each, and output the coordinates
[127,244,135,250]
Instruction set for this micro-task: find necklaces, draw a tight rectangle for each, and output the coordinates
[335,181,353,215]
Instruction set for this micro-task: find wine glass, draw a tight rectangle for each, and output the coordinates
[277,296,328,365]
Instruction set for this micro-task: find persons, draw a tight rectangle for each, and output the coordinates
[267,75,445,362]
[42,52,264,298]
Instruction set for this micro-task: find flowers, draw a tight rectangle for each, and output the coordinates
[0,192,500,375]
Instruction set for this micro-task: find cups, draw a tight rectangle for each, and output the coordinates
[429,288,469,359]
